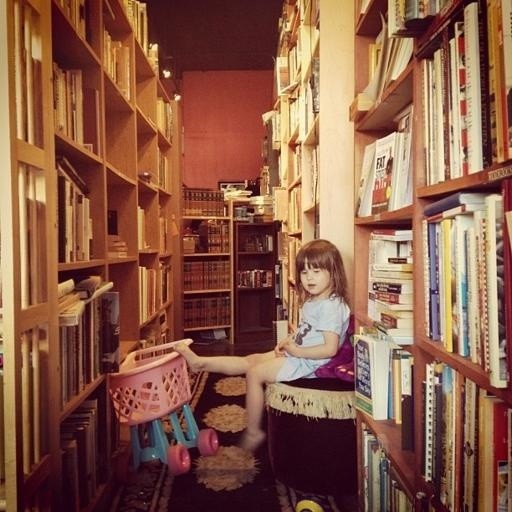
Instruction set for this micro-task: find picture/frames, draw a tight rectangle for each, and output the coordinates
[217,181,245,191]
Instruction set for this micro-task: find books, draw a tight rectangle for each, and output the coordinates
[183,187,274,345]
[350,0,512,511]
[2,2,173,511]
[261,0,319,346]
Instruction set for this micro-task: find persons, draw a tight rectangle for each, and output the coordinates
[172,240,350,458]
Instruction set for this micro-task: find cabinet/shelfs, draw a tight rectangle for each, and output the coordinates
[223,199,282,346]
[181,189,235,346]
